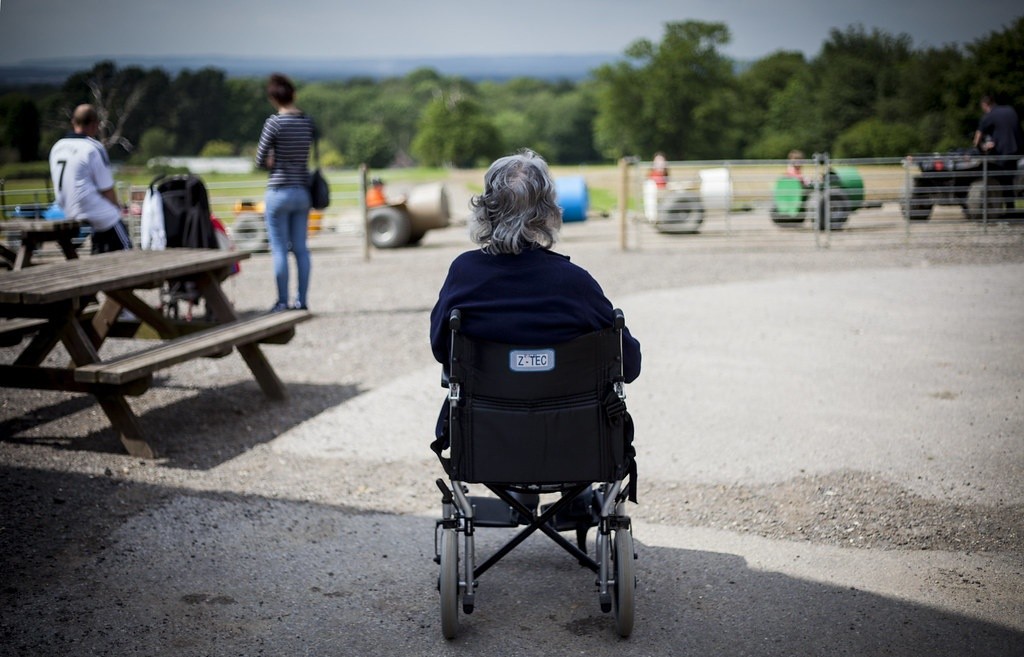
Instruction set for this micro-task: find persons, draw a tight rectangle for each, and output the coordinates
[785,150,844,229]
[427,148,643,515]
[645,155,675,190]
[253,73,313,311]
[49,104,133,256]
[973,96,1020,219]
[365,179,389,207]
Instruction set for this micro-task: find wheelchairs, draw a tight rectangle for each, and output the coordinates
[433,308,640,642]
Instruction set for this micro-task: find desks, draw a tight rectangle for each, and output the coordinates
[0,219,97,346]
[0,246,286,460]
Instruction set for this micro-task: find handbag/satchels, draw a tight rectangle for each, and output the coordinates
[140,184,167,252]
[307,171,329,210]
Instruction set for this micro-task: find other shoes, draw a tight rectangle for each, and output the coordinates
[506,484,539,511]
[556,483,592,520]
[271,302,288,312]
[115,307,136,322]
[291,301,307,310]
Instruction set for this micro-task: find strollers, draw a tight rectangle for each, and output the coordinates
[143,169,227,322]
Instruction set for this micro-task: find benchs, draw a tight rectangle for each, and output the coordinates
[74,310,319,388]
